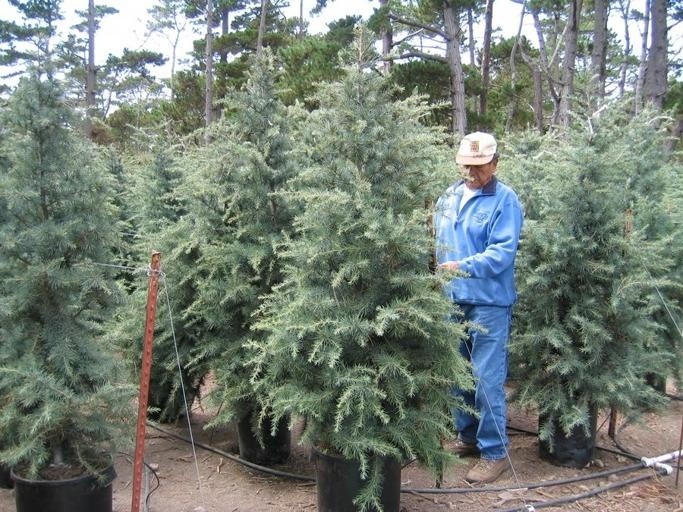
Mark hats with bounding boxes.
[454,131,497,166]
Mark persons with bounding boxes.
[427,131,522,486]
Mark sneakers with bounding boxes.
[443,441,480,457]
[466,457,514,485]
